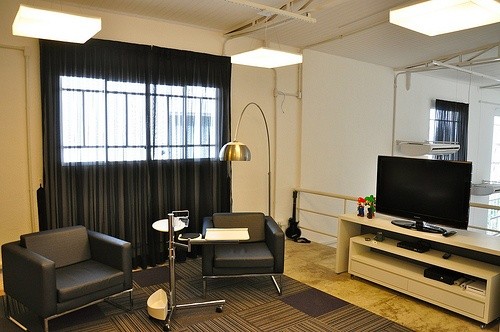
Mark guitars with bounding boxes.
[285,190,302,240]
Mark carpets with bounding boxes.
[0,258,423,332]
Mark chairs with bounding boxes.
[197,212,285,293]
[1,226,134,332]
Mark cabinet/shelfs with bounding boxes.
[347,234,500,328]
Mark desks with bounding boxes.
[334,211,500,321]
[159,228,251,332]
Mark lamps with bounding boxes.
[231,17,303,67]
[217,102,273,217]
[12,0,102,45]
[387,0,500,40]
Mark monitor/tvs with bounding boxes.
[375,155,471,236]
